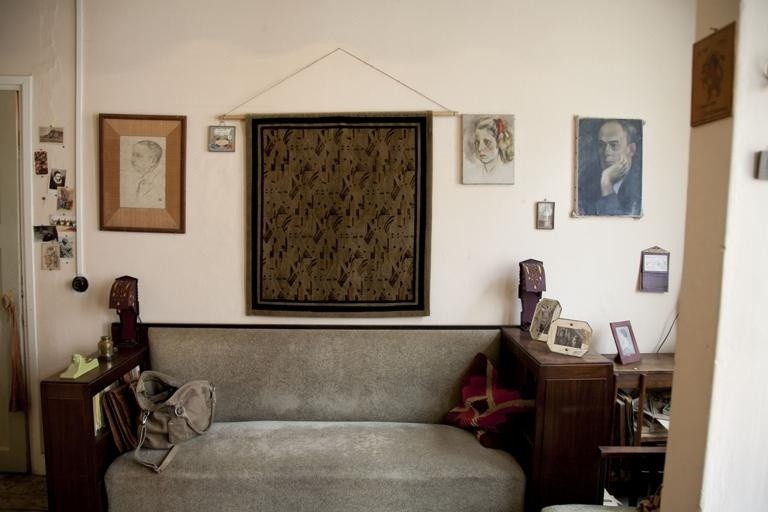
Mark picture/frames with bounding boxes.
[96,113,187,235]
[529,298,562,342]
[572,114,645,220]
[688,20,737,129]
[535,201,556,230]
[611,317,642,365]
[545,317,593,358]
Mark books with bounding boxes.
[616,388,670,446]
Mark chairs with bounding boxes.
[540,445,666,512]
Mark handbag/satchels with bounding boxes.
[134,369,216,470]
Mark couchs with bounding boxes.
[103,324,527,511]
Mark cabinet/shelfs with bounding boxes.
[38,341,152,512]
[601,353,675,496]
[500,328,614,511]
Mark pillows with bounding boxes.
[438,351,535,449]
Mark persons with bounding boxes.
[463,115,514,183]
[576,119,641,215]
[126,139,164,208]
[49,171,65,190]
[570,336,580,347]
[555,329,568,344]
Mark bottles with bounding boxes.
[98,335,113,359]
